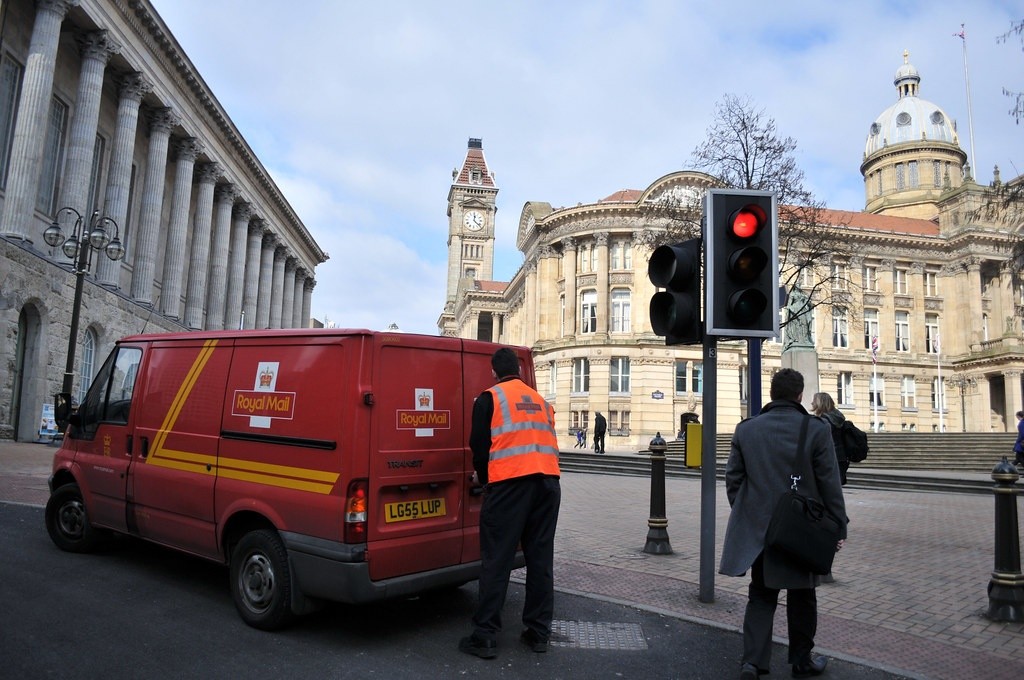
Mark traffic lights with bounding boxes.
[705,189,778,335]
[682,422,703,469]
[647,240,702,346]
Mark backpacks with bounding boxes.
[823,415,868,462]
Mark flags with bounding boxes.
[871,335,879,365]
[934,334,942,352]
[950,31,965,41]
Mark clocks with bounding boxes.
[462,209,487,231]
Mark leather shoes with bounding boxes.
[460,636,496,658]
[523,626,548,652]
[792,656,827,678]
[741,662,758,680]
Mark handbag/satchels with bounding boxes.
[767,493,842,574]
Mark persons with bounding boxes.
[809,392,850,528]
[1011,411,1024,469]
[593,409,606,454]
[459,348,561,658]
[718,370,850,680]
[574,426,587,450]
[782,282,816,355]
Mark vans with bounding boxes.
[43,327,527,633]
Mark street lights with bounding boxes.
[44,205,125,444]
